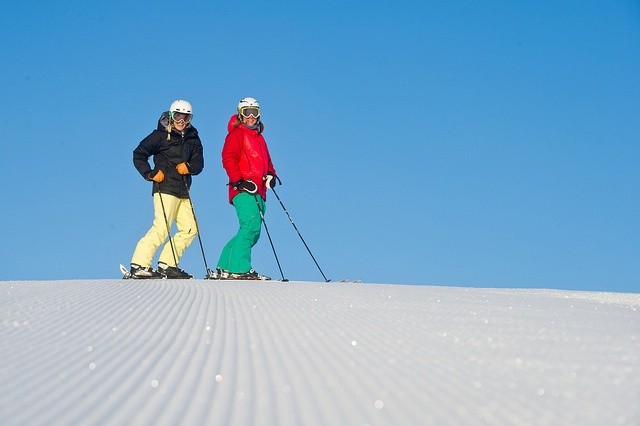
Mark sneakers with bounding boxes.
[216,267,229,277]
[157,262,192,277]
[231,268,270,280]
[130,263,159,277]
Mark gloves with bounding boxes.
[148,168,165,183]
[226,177,258,194]
[175,161,191,175]
[262,171,282,190]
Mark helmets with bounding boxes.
[170,99,192,114]
[237,97,259,111]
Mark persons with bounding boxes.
[216,97,276,278]
[130,100,203,279]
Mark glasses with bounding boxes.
[240,106,260,119]
[172,112,193,123]
